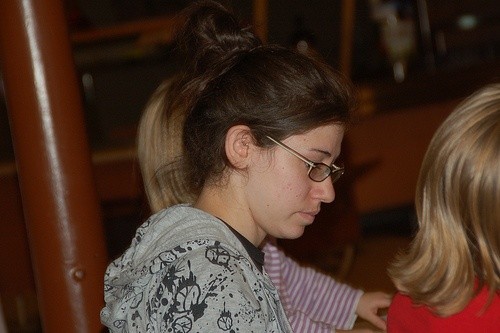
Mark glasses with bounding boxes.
[265,135,345,185]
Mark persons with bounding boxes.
[260,240,392,332]
[99,0,350,333]
[386,85,500,333]
